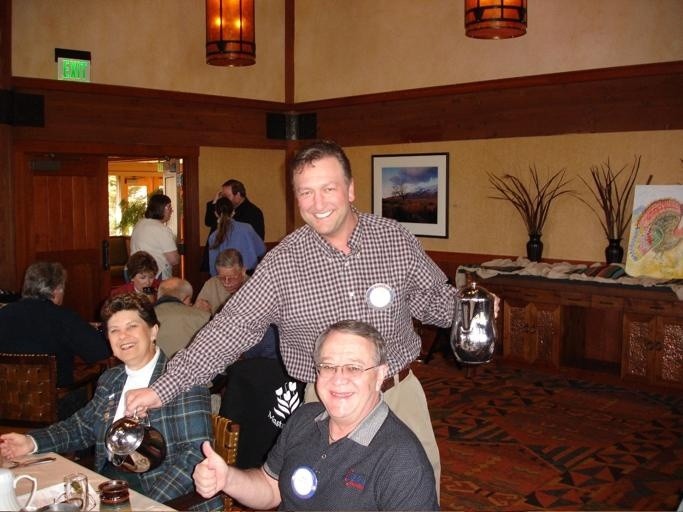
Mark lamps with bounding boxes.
[206,0,256,69]
[465,0,528,43]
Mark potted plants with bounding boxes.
[571,155,654,266]
[485,163,577,263]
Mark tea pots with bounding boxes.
[0,468,38,512]
[450,280,498,366]
[104,407,166,475]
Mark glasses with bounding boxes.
[312,362,379,375]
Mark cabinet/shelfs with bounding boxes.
[622,284,681,400]
[502,276,561,384]
[561,281,622,389]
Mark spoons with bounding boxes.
[3,455,56,470]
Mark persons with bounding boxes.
[130,195,181,280]
[195,249,276,360]
[213,323,307,470]
[101,252,159,319]
[124,138,500,511]
[149,278,214,358]
[192,319,440,511]
[0,292,226,511]
[208,198,268,276]
[204,178,265,249]
[0,262,113,387]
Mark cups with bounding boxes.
[64,473,96,512]
[177,241,186,255]
[98,480,132,512]
[142,286,158,295]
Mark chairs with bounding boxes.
[1,351,59,425]
[165,414,240,512]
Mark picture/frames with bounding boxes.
[370,152,450,239]
